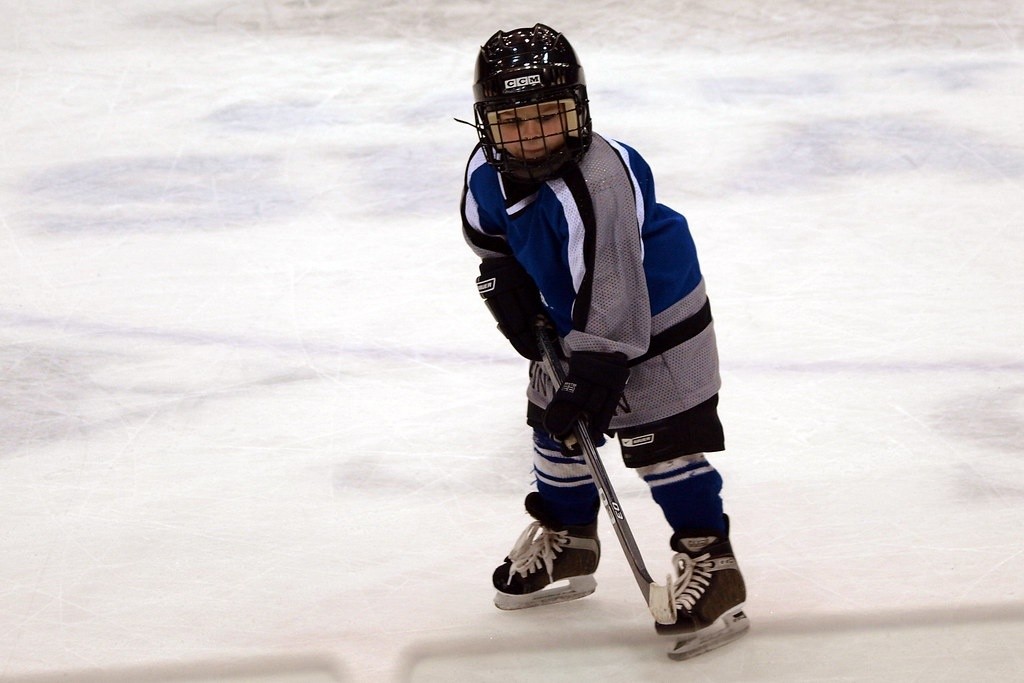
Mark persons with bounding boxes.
[453,22,749,661]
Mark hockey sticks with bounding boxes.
[535,326,679,627]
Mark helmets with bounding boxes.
[454,23,592,185]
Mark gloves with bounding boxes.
[476,255,558,361]
[542,351,631,457]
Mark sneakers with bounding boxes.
[655,513,750,660]
[491,492,601,610]
